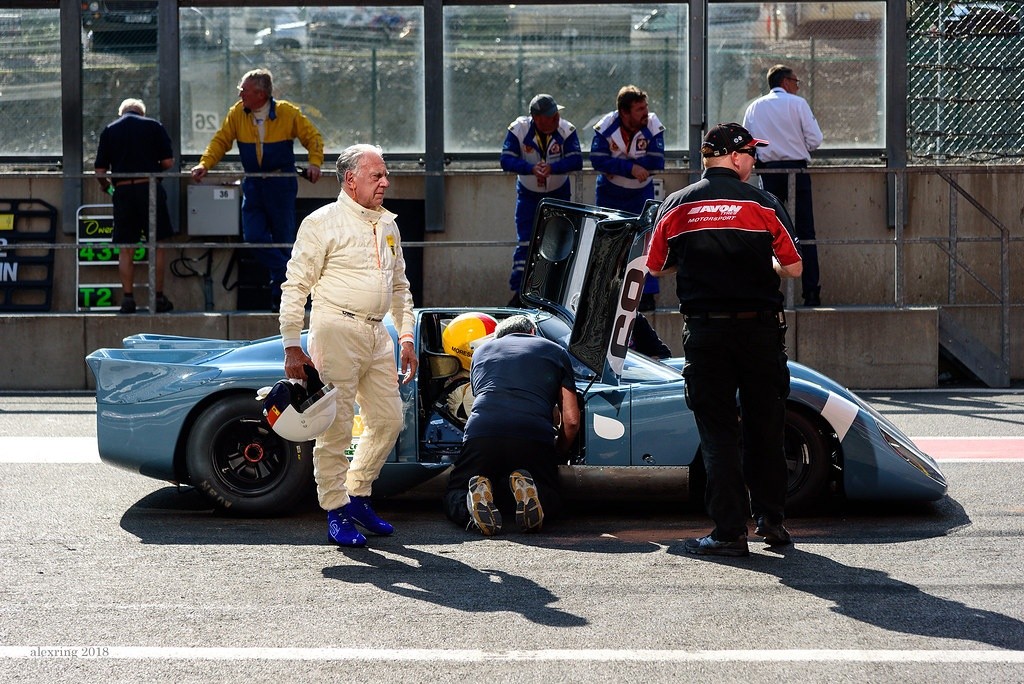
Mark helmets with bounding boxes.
[442,310,499,371]
[257,380,337,442]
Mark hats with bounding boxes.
[528,94,565,116]
[701,122,770,157]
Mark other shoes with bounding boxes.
[509,469,544,531]
[157,297,174,313]
[507,293,527,308]
[465,475,502,535]
[639,302,656,312]
[803,292,821,307]
[119,300,138,313]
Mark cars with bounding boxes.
[79,3,519,52]
[632,4,792,45]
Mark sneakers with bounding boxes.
[754,524,795,546]
[328,505,366,548]
[685,528,751,555]
[346,498,395,535]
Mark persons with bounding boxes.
[95,98,174,312]
[191,70,325,311]
[279,144,419,548]
[444,315,581,534]
[442,311,498,422]
[591,86,665,311]
[646,122,802,553]
[743,66,822,307]
[501,94,584,307]
[629,312,671,360]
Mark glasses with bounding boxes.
[786,77,801,85]
[737,148,758,160]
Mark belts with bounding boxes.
[342,311,382,322]
[687,311,759,320]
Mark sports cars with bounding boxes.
[86,199,949,519]
